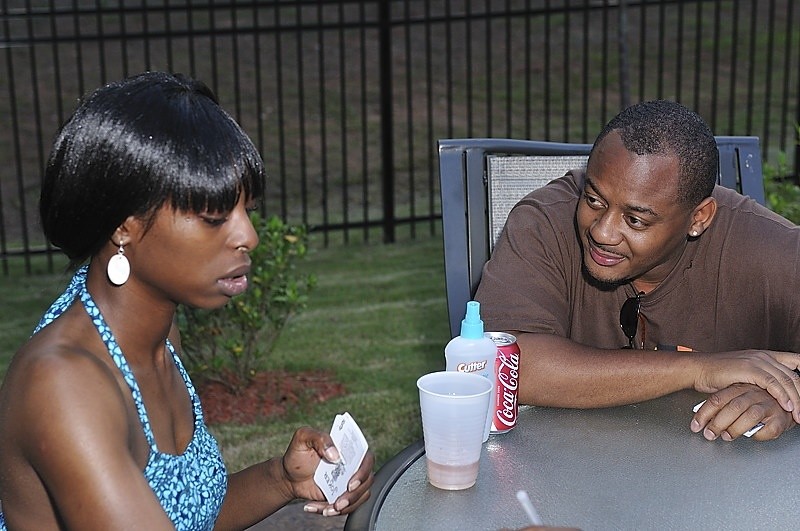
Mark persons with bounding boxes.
[0,73,374,531]
[471,100,800,441]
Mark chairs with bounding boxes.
[438,136,765,339]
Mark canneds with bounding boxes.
[482,332,520,435]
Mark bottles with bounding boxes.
[444,301,497,444]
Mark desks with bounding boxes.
[343,406,800,531]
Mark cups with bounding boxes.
[416,371,494,490]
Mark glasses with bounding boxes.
[619,291,644,350]
